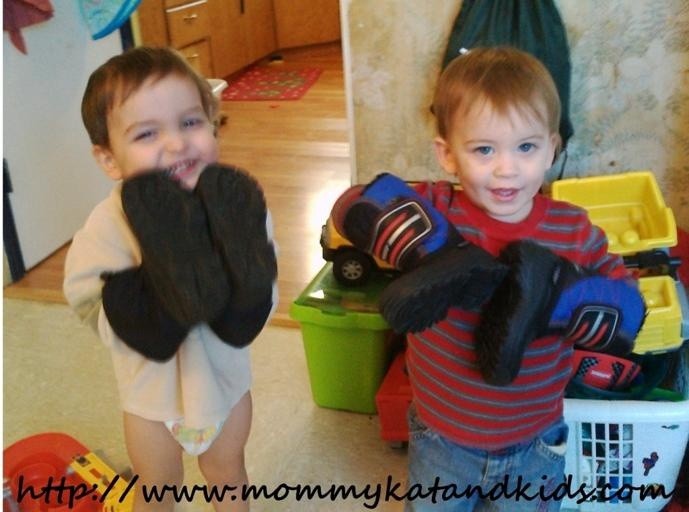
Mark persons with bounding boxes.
[63,43,278,512]
[332,44,646,511]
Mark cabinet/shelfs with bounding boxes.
[273,1,342,50]
[129,1,215,82]
[208,0,274,82]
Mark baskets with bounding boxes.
[556,246,689,512]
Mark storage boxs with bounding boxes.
[287,261,391,413]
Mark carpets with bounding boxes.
[221,64,325,100]
[4,296,410,512]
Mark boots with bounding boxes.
[99,167,232,364]
[342,172,510,336]
[472,238,647,387]
[193,160,278,349]
